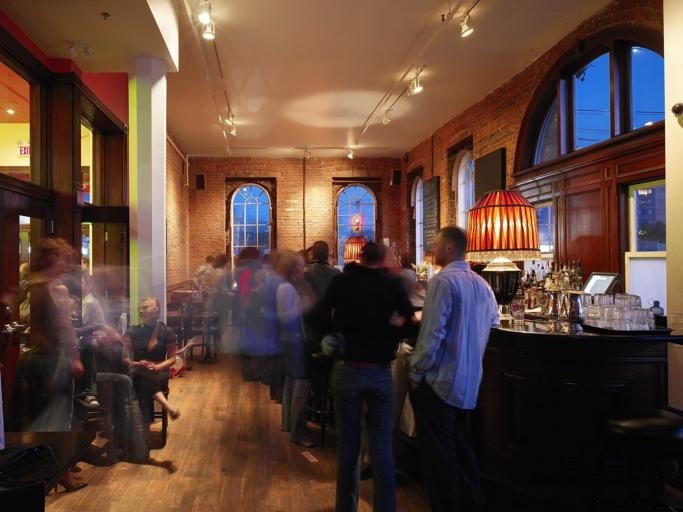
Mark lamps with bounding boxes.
[225,112,237,137]
[199,3,215,39]
[463,190,542,326]
[460,21,473,39]
[343,235,369,264]
[380,77,423,126]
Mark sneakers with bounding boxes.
[294,435,315,448]
[171,409,181,420]
[361,460,372,481]
[199,354,213,364]
[77,395,99,409]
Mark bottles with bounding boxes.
[650,300,664,315]
[522,257,583,292]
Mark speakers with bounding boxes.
[391,169,401,186]
[195,173,206,192]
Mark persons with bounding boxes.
[193,241,343,449]
[305,241,423,510]
[404,227,500,510]
[22,239,179,495]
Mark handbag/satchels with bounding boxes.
[0,442,57,487]
[320,332,344,358]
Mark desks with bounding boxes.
[171,290,235,339]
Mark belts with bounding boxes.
[350,361,383,368]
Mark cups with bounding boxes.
[511,304,524,327]
[579,293,655,331]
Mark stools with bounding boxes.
[305,352,346,450]
[606,406,682,512]
[151,386,171,447]
[167,301,220,362]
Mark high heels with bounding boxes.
[54,476,88,493]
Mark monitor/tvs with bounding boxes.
[580,271,624,299]
